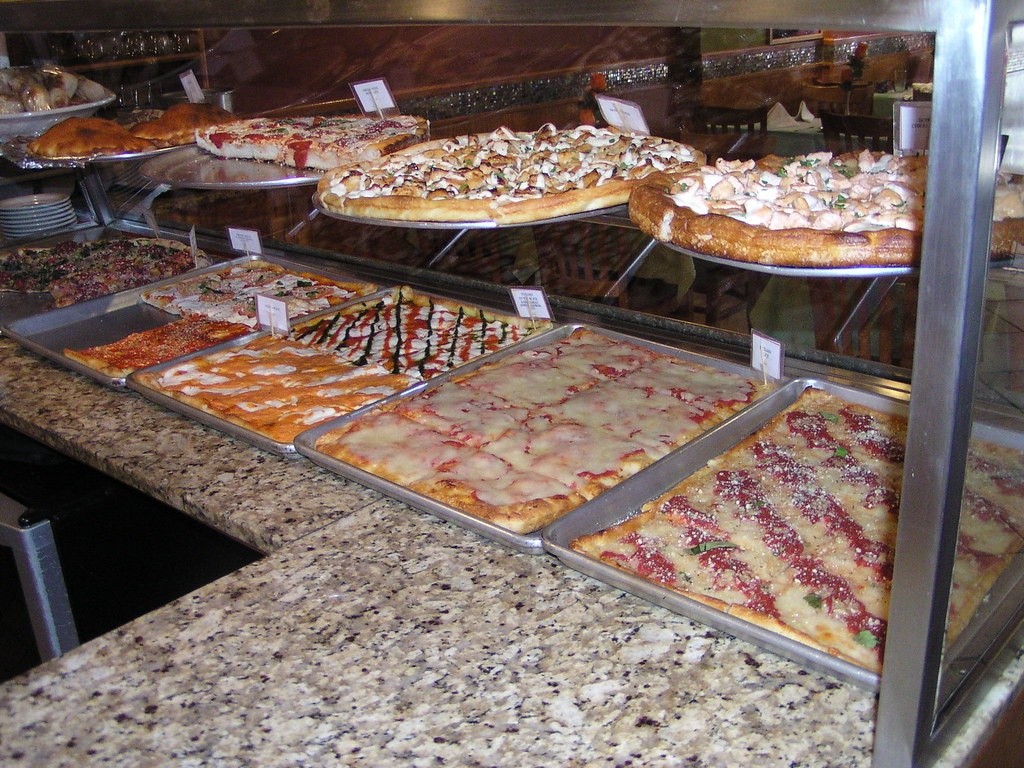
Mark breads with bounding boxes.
[0,65,106,115]
[29,102,240,157]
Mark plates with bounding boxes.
[1,194,77,242]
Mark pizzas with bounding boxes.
[59,259,555,443]
[310,326,768,537]
[629,150,1024,267]
[570,385,1024,680]
[193,114,431,171]
[0,236,211,307]
[314,124,706,225]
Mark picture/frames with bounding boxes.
[767,29,824,46]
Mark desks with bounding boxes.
[653,129,919,168]
[872,85,915,117]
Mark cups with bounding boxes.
[894,68,908,94]
[52,24,197,64]
[122,81,163,112]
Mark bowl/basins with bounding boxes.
[163,88,235,121]
[1,66,116,138]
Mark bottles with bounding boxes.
[822,38,835,62]
[822,65,831,83]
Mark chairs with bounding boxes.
[528,78,1008,371]
